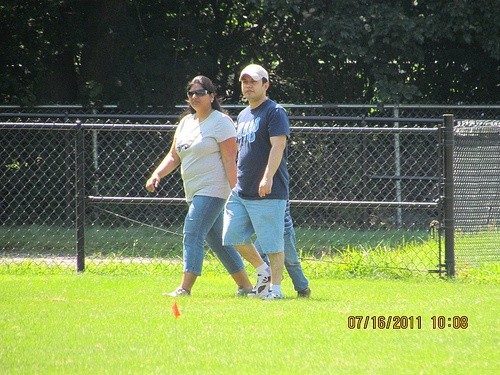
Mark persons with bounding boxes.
[145,76,255,300]
[220,64,290,301]
[254,198,312,298]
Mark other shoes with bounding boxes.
[297,286,311,297]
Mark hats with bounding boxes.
[238,63,269,81]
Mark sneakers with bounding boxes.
[235,287,254,297]
[247,273,285,301]
[163,287,190,298]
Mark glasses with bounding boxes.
[187,89,211,96]
